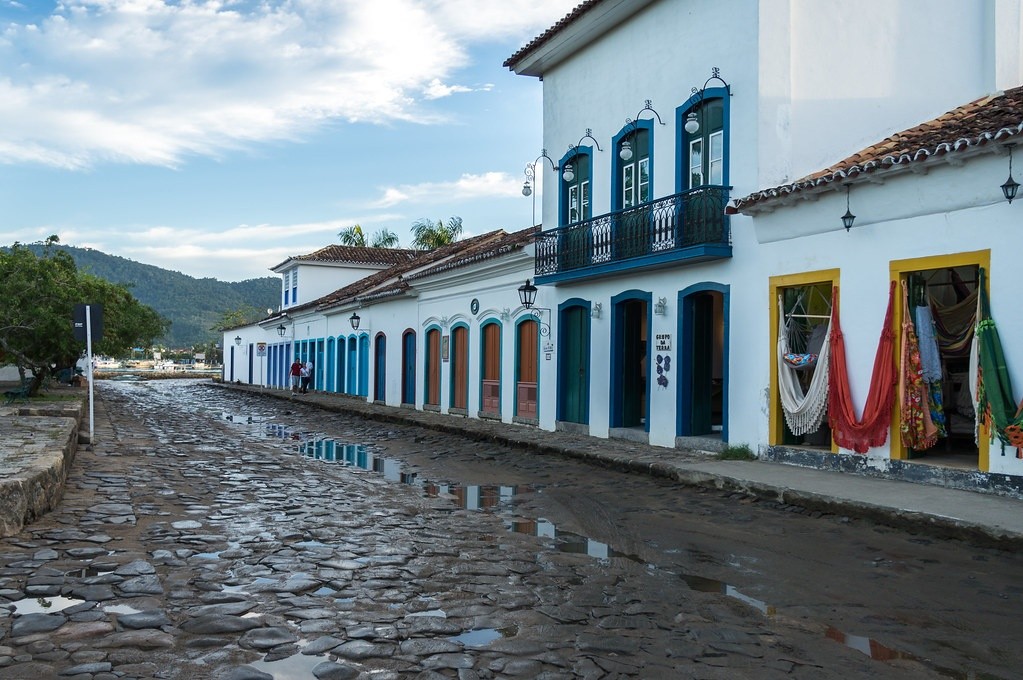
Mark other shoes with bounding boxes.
[303,393,307,396]
[292,392,297,396]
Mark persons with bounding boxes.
[288,358,312,396]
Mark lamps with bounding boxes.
[590,301,603,320]
[620,100,666,160]
[840,183,856,232]
[349,312,371,346]
[521,148,560,196]
[1000,142,1021,204]
[517,279,551,340]
[499,307,510,322]
[234,336,248,355]
[654,297,668,317]
[684,66,733,134]
[276,324,295,340]
[563,128,603,182]
[438,315,448,328]
